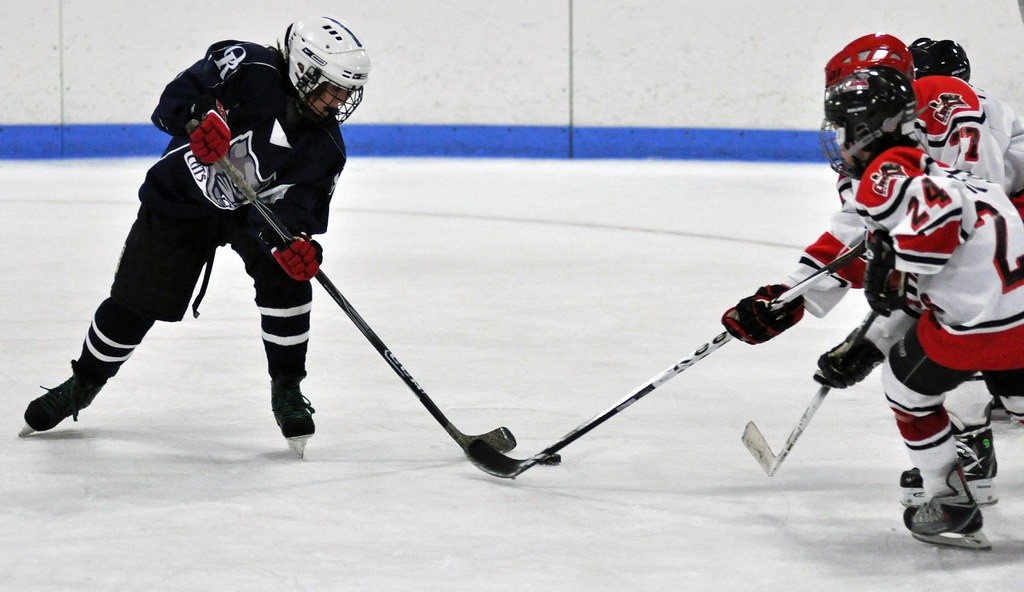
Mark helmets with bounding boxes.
[821,65,918,181]
[284,16,370,128]
[907,37,971,82]
[824,33,914,90]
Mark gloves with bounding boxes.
[813,327,886,389]
[257,229,323,282]
[863,229,896,318]
[185,92,232,166]
[721,284,806,345]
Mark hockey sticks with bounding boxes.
[465,233,883,481]
[739,269,902,478]
[183,118,518,458]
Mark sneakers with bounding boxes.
[18,377,91,438]
[903,457,992,551]
[980,394,1024,431]
[900,417,998,509]
[271,379,315,459]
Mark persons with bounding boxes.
[814,62,1024,549]
[722,34,1023,508]
[20,16,370,458]
